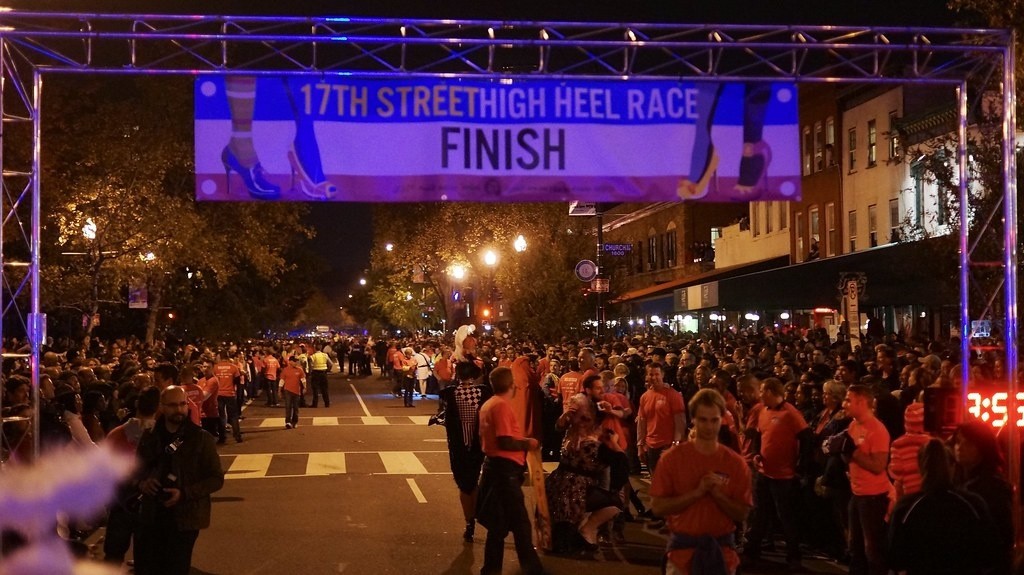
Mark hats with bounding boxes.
[648,347,665,356]
[148,363,178,378]
[917,355,942,369]
[290,356,299,362]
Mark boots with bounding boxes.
[629,492,645,513]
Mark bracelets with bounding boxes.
[672,440,681,445]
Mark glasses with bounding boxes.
[162,402,189,407]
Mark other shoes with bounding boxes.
[578,532,598,551]
[789,559,800,570]
[624,510,633,522]
[285,422,293,428]
[309,405,317,408]
[216,440,225,444]
[659,525,670,534]
[325,401,330,407]
[463,523,475,542]
[742,556,759,566]
[644,521,662,530]
[234,436,242,442]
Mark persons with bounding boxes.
[0,311,1024,575]
